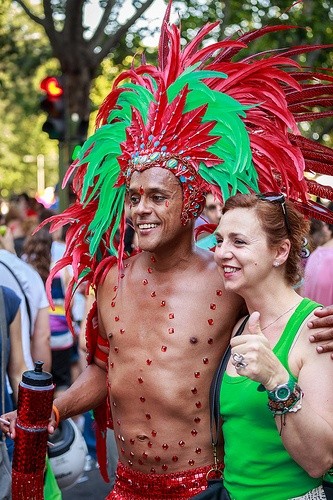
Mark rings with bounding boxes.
[233,353,246,369]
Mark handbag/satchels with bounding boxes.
[0,442,13,500]
[190,479,231,500]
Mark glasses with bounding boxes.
[255,192,291,239]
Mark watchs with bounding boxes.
[266,376,295,401]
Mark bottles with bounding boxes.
[10,361,55,499]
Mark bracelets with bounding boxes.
[53,406,59,428]
[268,384,304,436]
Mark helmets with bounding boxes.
[47,417,88,489]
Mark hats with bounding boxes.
[30,0,333,335]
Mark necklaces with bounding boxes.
[261,303,300,331]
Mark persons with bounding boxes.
[212,194,333,500]
[301,237,333,307]
[0,154,333,500]
[1,183,99,500]
[195,194,223,248]
